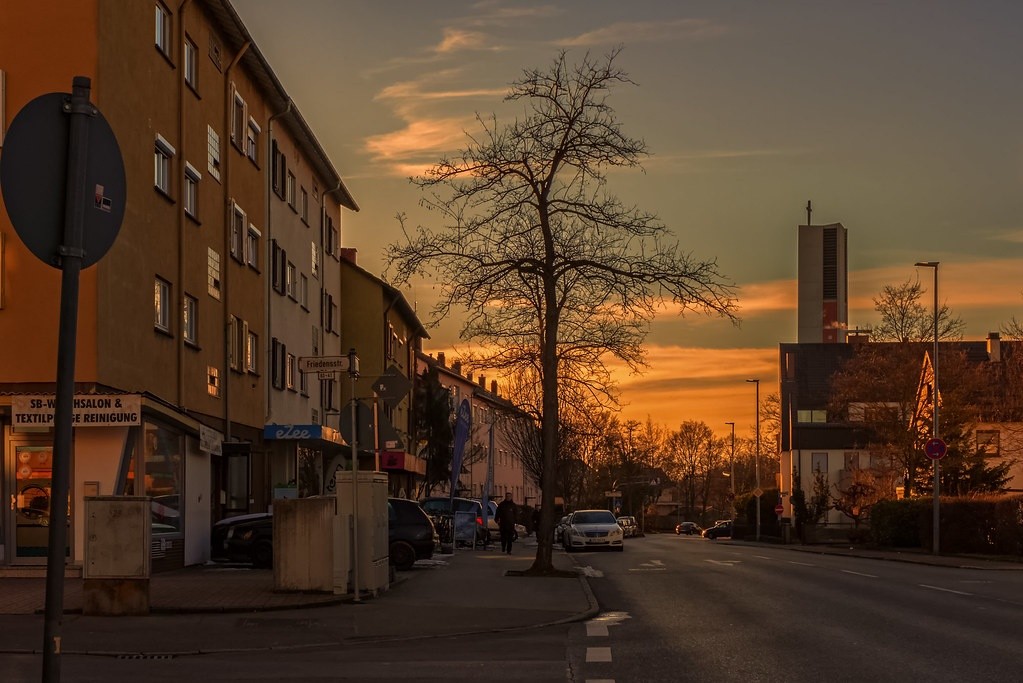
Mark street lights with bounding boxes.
[611,477,660,518]
[745,378,764,542]
[724,421,735,526]
[914,260,942,556]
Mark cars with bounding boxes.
[557,516,567,544]
[561,513,573,547]
[417,495,488,548]
[486,500,528,544]
[211,493,441,573]
[564,509,625,551]
[702,520,731,540]
[676,522,702,536]
[616,516,639,538]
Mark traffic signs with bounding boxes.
[354,356,360,382]
[297,355,351,374]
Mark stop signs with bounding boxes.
[775,504,784,515]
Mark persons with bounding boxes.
[530,509,540,543]
[493,492,520,556]
[792,506,803,542]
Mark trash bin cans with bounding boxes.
[801,518,816,545]
